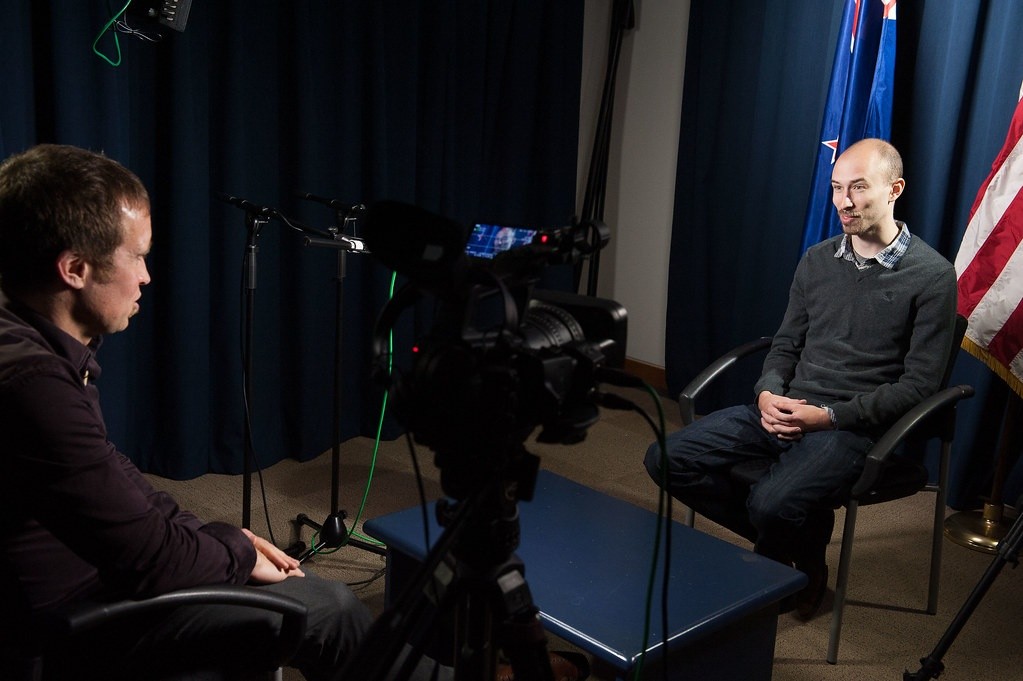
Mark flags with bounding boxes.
[953,80,1023,398]
[802,1,897,254]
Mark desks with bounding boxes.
[363,466,808,681]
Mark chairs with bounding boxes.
[0,585,311,681]
[679,313,974,665]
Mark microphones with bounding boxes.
[298,235,370,256]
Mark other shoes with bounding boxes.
[753,545,827,622]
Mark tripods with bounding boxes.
[308,495,557,681]
[295,247,393,570]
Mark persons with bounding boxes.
[0,144,368,681]
[643,137,958,625]
[478,227,516,259]
